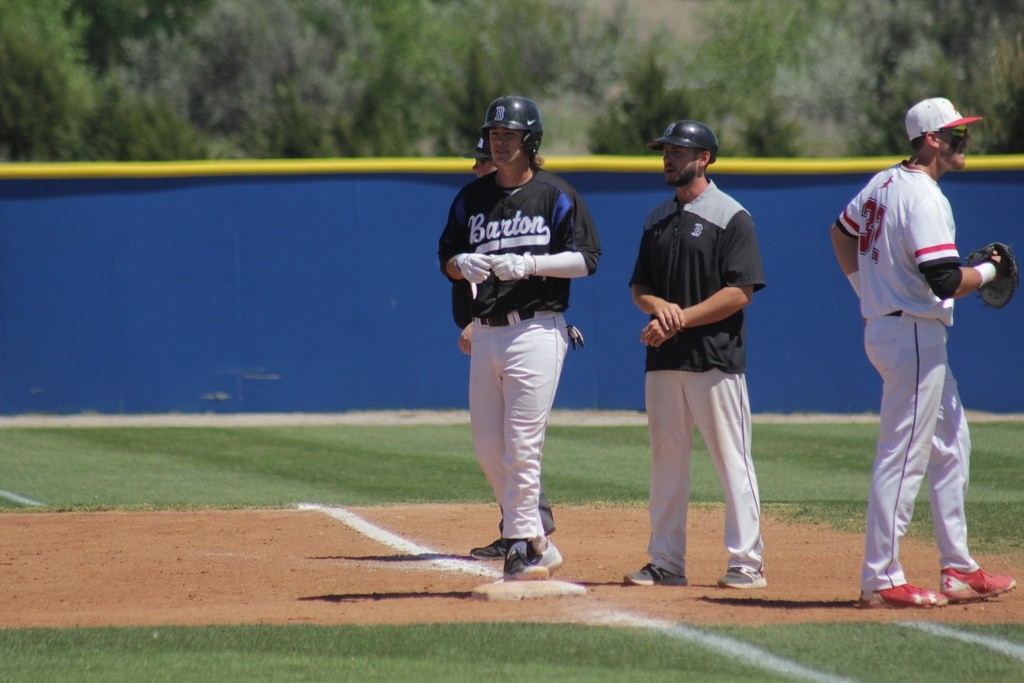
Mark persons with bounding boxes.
[830,96,1018,611]
[437,95,603,583]
[623,120,768,591]
[450,128,555,539]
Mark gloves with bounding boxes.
[566,324,585,349]
[487,253,536,280]
[455,253,495,284]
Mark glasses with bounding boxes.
[923,125,968,139]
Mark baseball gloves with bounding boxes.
[966,242,1021,310]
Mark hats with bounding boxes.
[462,136,492,159]
[905,98,984,142]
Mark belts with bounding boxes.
[888,310,902,317]
[472,311,534,328]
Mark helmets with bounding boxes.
[479,96,542,154]
[647,121,718,163]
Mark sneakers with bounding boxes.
[623,564,687,587]
[496,539,562,585]
[938,564,1017,605]
[859,577,947,608]
[718,563,768,589]
[469,538,506,560]
